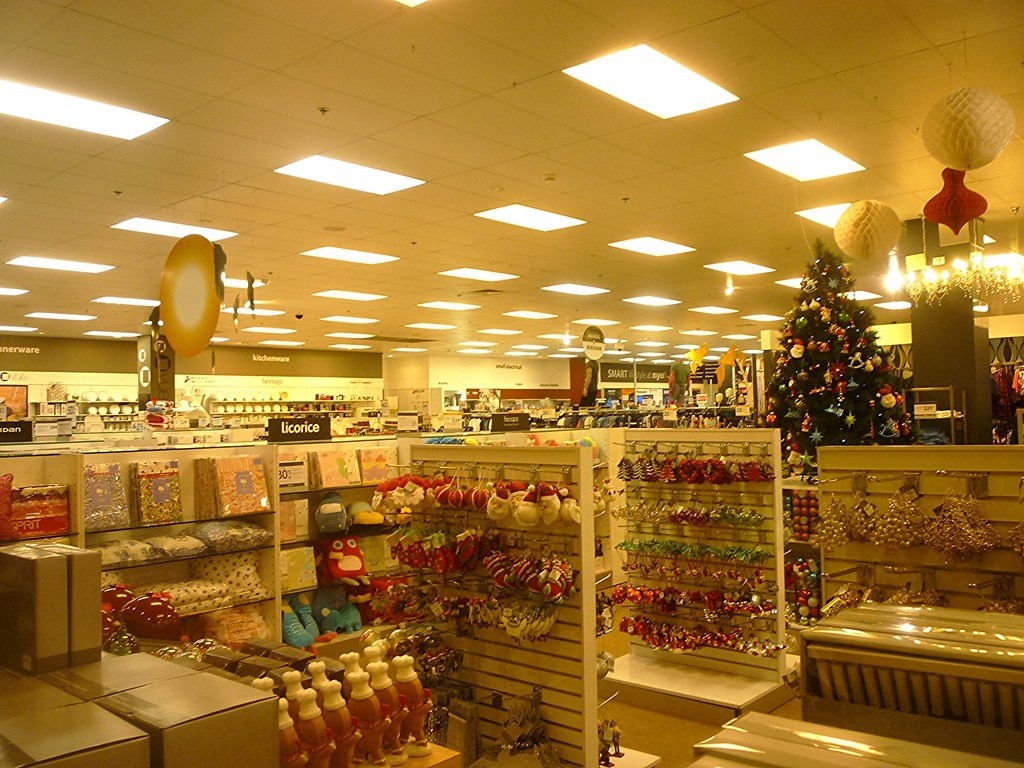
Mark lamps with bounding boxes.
[906,236,1023,313]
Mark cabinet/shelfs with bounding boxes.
[0,451,82,549]
[782,476,822,655]
[277,438,411,666]
[897,378,974,447]
[80,445,279,658]
[31,394,537,441]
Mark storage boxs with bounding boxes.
[685,706,1024,768]
[0,539,280,768]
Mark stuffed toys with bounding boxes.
[281,473,616,659]
